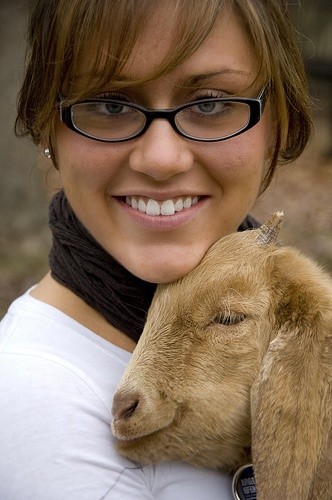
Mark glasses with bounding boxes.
[56,80,272,143]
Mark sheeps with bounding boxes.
[109,209,332,500]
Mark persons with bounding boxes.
[0,0,315,500]
